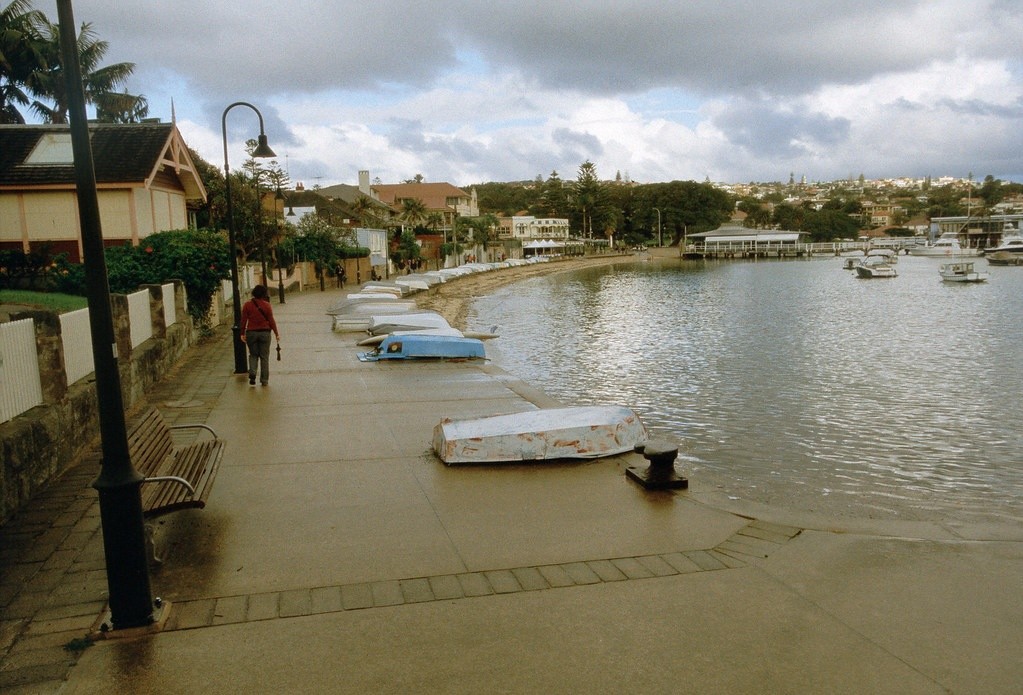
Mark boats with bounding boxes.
[854,255,899,278]
[321,257,549,363]
[866,249,899,264]
[982,237,1023,256]
[430,404,652,463]
[907,237,984,257]
[938,260,989,283]
[843,252,867,270]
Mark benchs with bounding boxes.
[128,405,227,568]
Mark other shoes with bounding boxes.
[262,382,268,386]
[248,372,256,385]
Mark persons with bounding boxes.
[398,258,416,275]
[498,251,507,261]
[241,285,280,385]
[335,264,344,289]
[371,265,377,281]
[464,253,476,264]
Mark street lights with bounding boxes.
[222,101,273,378]
[274,194,297,303]
[315,208,334,290]
[256,168,288,303]
[652,207,662,247]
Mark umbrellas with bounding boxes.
[523,239,561,256]
